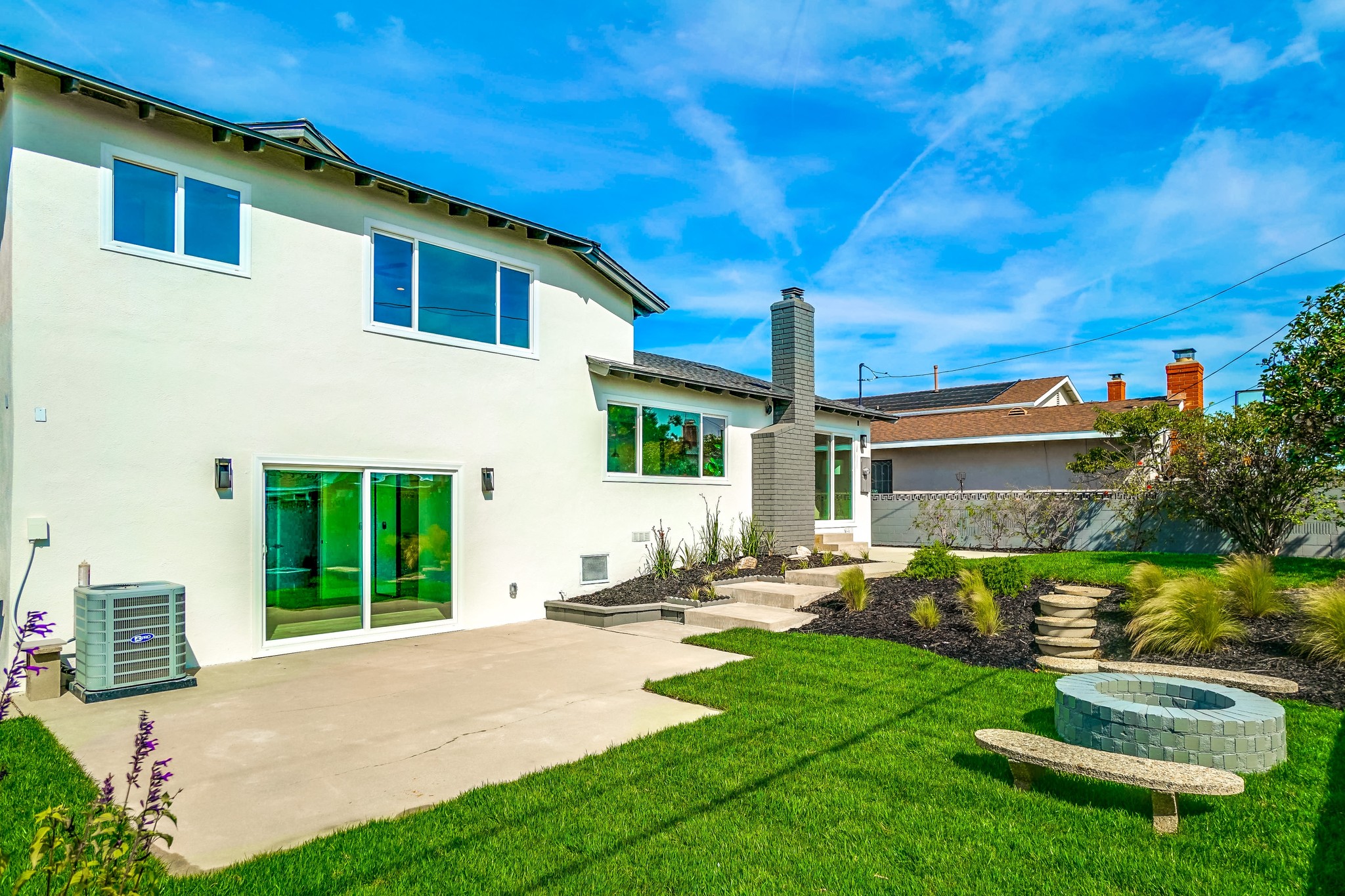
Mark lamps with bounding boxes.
[510,582,518,595]
[860,434,867,449]
[214,457,233,489]
[481,467,494,491]
[766,405,773,414]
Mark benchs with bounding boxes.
[975,729,1246,834]
[1097,661,1299,693]
